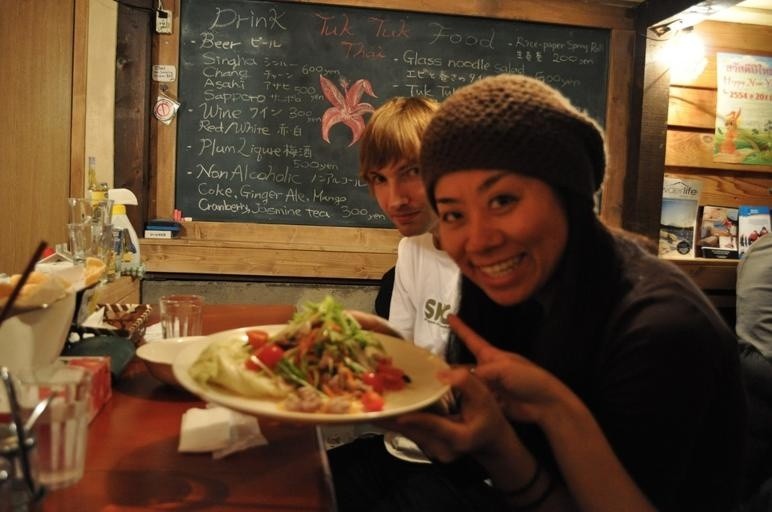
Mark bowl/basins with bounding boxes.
[135,335,205,386]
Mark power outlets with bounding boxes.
[154,8,175,34]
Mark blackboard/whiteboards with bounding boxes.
[157,0,635,255]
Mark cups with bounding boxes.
[68,183,125,285]
[16,365,92,485]
[0,423,41,512]
[159,292,206,339]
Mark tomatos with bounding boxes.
[362,355,410,412]
[244,329,285,371]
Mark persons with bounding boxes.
[734,227,772,360]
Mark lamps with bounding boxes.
[665,24,711,87]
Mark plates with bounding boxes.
[173,323,452,426]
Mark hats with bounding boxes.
[420,74,606,216]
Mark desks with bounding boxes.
[28,300,338,511]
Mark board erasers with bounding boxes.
[147,219,182,232]
